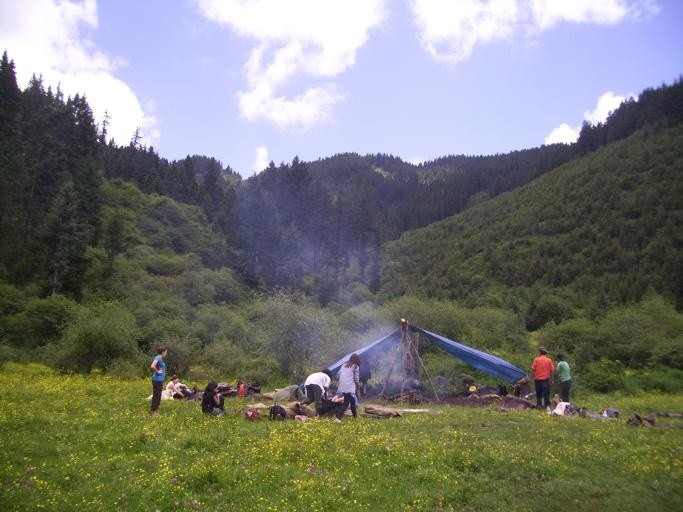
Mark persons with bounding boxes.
[529,347,554,408]
[555,353,570,403]
[300,369,332,420]
[200,381,225,415]
[333,393,358,409]
[150,346,167,417]
[335,353,360,422]
[167,375,192,399]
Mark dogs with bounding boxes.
[268,401,287,421]
